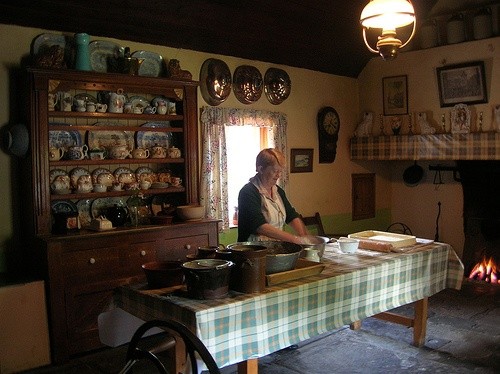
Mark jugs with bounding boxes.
[108,93,125,113]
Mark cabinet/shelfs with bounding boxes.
[8,65,224,365]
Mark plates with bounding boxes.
[73,91,171,109]
[89,41,124,73]
[33,33,75,70]
[50,193,177,231]
[51,168,155,186]
[49,122,174,147]
[131,50,163,77]
[152,183,168,189]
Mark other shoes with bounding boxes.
[284,345,298,350]
[276,349,283,355]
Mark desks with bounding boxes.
[112,231,465,374]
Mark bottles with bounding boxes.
[74,33,92,71]
[197,246,220,259]
[157,102,167,114]
[304,248,321,262]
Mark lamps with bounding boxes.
[360,0,417,62]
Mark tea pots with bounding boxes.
[100,205,129,227]
[124,103,143,114]
[144,105,156,114]
[49,146,181,161]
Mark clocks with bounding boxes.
[317,106,341,164]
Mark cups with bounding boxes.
[76,101,107,112]
[170,177,182,186]
[125,181,152,190]
[49,94,73,111]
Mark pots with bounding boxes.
[404,161,424,183]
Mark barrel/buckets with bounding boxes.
[184,245,267,300]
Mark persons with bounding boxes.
[237,148,318,351]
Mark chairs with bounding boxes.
[299,211,347,239]
[128,320,220,374]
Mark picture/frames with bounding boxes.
[290,148,314,173]
[382,74,409,116]
[436,61,488,108]
[352,173,375,221]
[450,103,470,134]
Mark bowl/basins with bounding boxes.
[176,205,206,222]
[142,254,197,290]
[227,237,360,275]
